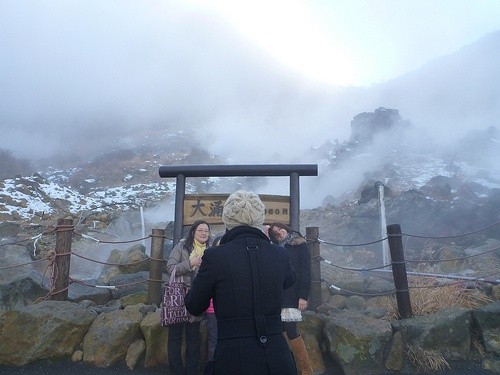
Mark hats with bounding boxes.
[222,190,265,231]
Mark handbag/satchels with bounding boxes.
[160,265,192,327]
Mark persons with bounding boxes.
[184,189,297,375]
[268,222,314,375]
[204,236,222,361]
[165,219,211,375]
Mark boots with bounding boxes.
[282,331,313,375]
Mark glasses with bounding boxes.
[196,230,209,233]
